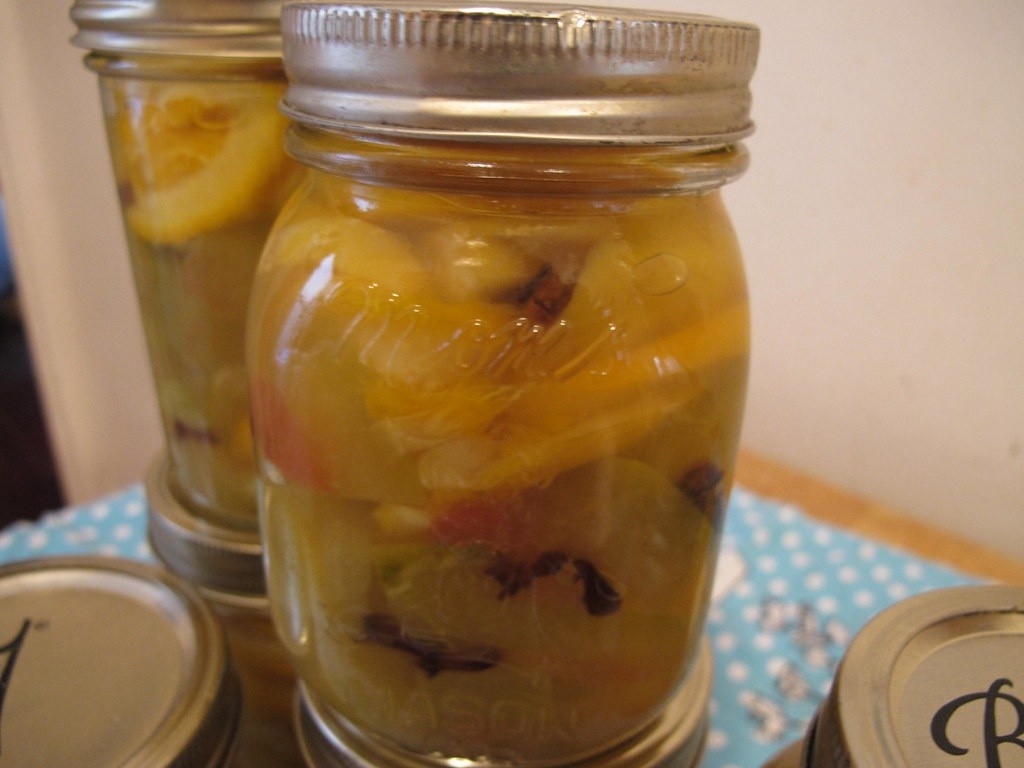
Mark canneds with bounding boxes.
[1,0,1024,768]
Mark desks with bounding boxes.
[0,449,1023,767]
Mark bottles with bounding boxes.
[241,1,762,768]
[66,0,310,542]
[139,445,302,768]
[0,554,247,768]
[760,582,1024,767]
